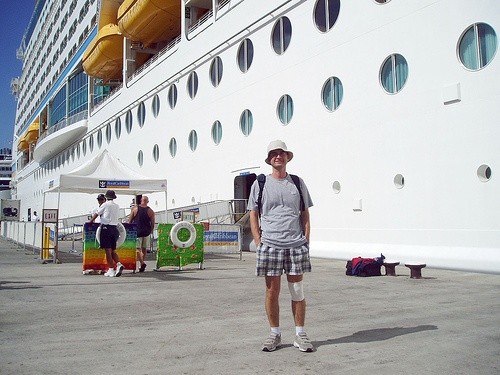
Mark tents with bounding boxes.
[41,149,168,263]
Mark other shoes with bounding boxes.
[139,264,147,273]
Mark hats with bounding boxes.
[96,194,105,199]
[265,140,293,163]
[104,190,117,199]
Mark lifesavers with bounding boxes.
[96,222,126,249]
[170,221,197,248]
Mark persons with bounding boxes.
[32,211,40,221]
[130,199,135,212]
[127,196,155,273]
[85,191,124,277]
[92,194,106,224]
[249,141,313,352]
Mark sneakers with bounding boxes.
[262,332,282,352]
[104,270,115,277]
[294,332,313,352]
[115,264,124,277]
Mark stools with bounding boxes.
[383,261,400,275]
[405,264,426,279]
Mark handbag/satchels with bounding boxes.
[353,257,377,268]
[346,260,352,275]
[353,256,386,276]
